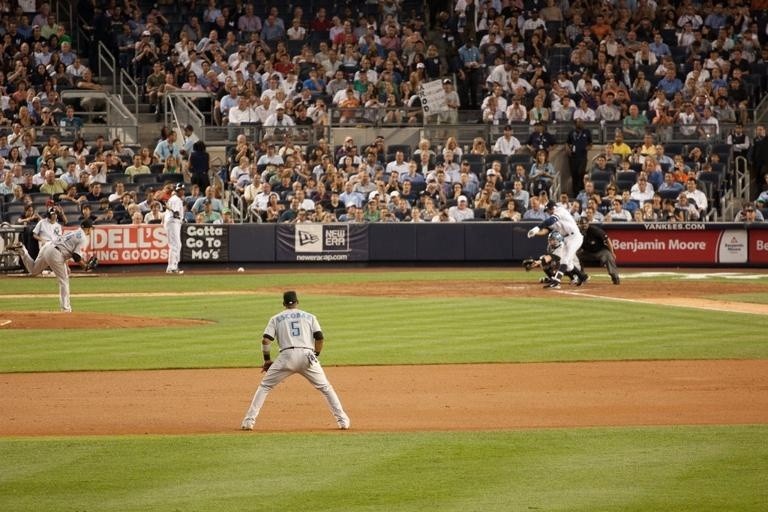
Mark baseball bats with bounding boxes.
[158,198,175,213]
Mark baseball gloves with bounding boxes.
[83,255,98,272]
[523,258,534,270]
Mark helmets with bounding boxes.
[174,182,186,192]
[548,231,563,242]
[576,216,590,235]
[48,207,56,215]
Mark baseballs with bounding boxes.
[238,267,244,273]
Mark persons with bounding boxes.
[2,1,767,222]
[7,208,95,312]
[240,290,351,430]
[160,183,187,275]
[525,201,619,289]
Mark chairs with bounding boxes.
[0,0,768,226]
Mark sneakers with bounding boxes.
[538,273,588,289]
[166,269,184,274]
[5,242,22,251]
[611,278,620,285]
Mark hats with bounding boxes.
[80,219,95,230]
[202,199,211,205]
[543,201,556,212]
[221,207,231,214]
[284,291,297,305]
[457,195,467,203]
[487,168,496,176]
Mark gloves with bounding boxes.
[173,210,179,219]
[528,226,540,239]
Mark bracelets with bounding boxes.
[315,351,320,357]
[261,344,272,352]
[264,354,271,361]
